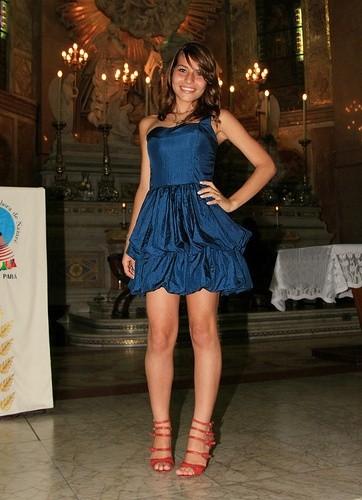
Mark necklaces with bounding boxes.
[169,107,195,124]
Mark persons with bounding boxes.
[122,42,277,480]
[120,104,136,140]
[254,91,267,138]
[107,23,127,67]
[61,74,78,135]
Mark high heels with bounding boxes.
[149,419,175,473]
[175,416,217,479]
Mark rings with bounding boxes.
[213,193,216,198]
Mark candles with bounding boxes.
[122,201,126,227]
[275,205,279,229]
[56,43,307,139]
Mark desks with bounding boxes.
[269,243,362,326]
